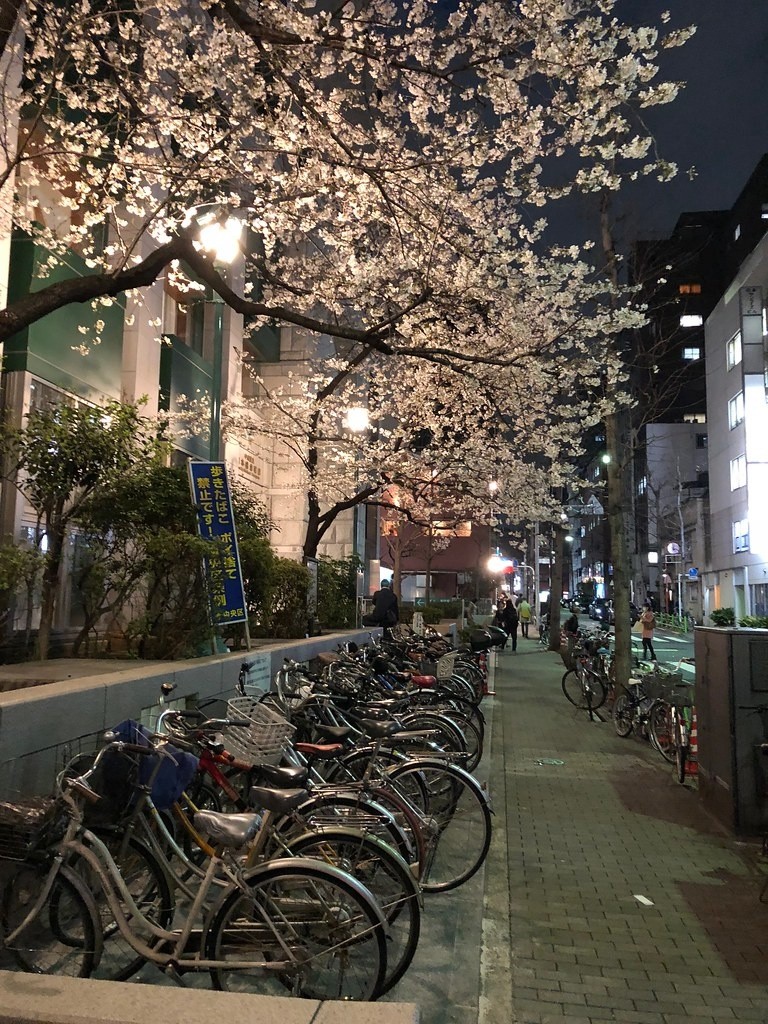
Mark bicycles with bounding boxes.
[0,620,510,1003]
[559,626,696,785]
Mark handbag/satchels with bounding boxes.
[644,614,657,630]
[105,719,192,807]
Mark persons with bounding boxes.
[371,579,400,624]
[490,590,612,652]
[639,603,658,661]
[644,596,685,616]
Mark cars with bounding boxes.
[607,600,640,627]
[588,598,612,622]
[570,593,596,614]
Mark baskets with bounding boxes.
[0,757,67,862]
[643,671,694,706]
[60,733,138,821]
[220,695,295,766]
[185,690,228,730]
[559,646,579,671]
